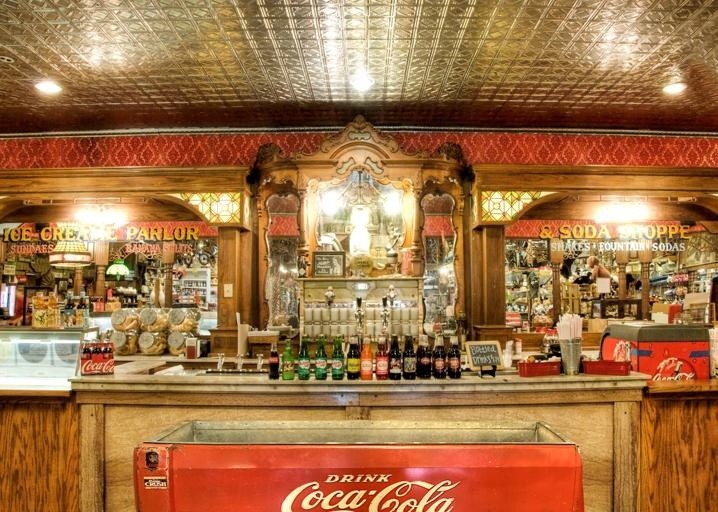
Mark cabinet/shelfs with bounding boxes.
[1,325,100,397]
[294,276,423,352]
[182,268,211,309]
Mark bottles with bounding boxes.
[331,334,344,380]
[184,281,206,287]
[552,330,560,354]
[282,339,295,380]
[360,337,373,380]
[138,332,167,356]
[167,304,200,332]
[376,338,388,380]
[298,335,310,380]
[416,334,431,378]
[110,333,137,355]
[268,341,279,380]
[314,334,328,380]
[76,292,86,327]
[432,335,446,379]
[140,307,168,332]
[389,334,402,380]
[47,292,60,327]
[346,338,361,380]
[34,292,46,327]
[166,333,194,355]
[515,338,522,355]
[91,339,103,375]
[81,339,91,376]
[403,334,416,380]
[447,335,461,379]
[109,309,139,331]
[63,291,76,328]
[102,339,114,374]
[543,331,552,353]
[91,295,104,312]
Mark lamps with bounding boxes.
[48,239,91,269]
[106,260,129,281]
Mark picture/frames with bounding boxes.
[311,251,346,277]
[464,340,505,372]
